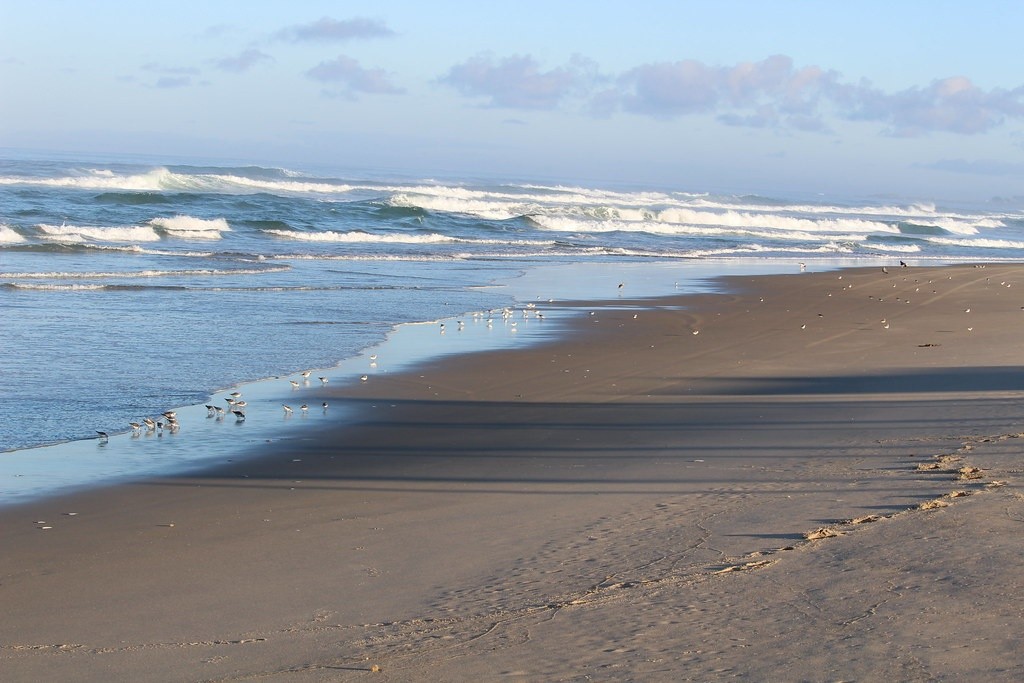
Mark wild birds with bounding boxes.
[759,260,1011,330]
[589,282,700,336]
[440,295,553,330]
[93,354,378,440]
